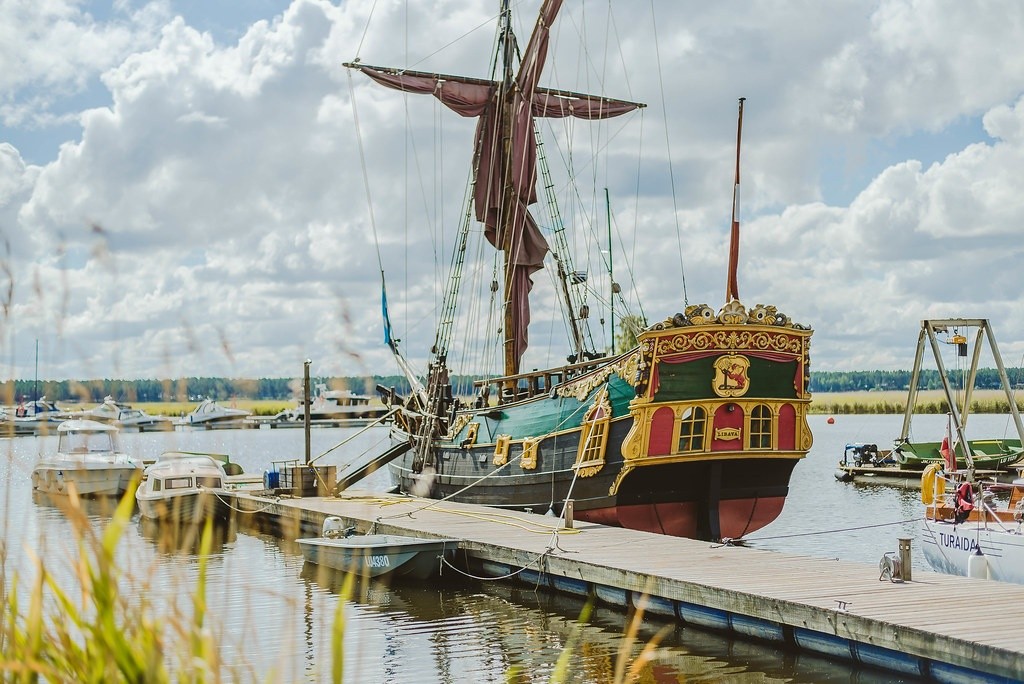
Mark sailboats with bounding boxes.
[341,0,817,546]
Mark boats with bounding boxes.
[921,463,1024,584]
[135,453,237,525]
[26,419,144,497]
[293,517,462,582]
[0,385,388,435]
[834,438,1024,492]
